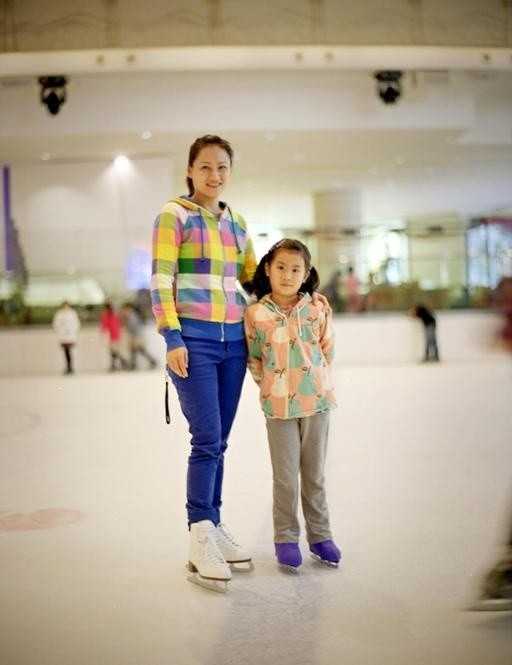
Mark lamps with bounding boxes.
[38,74,68,116]
[375,69,401,103]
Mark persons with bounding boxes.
[247,236,344,568]
[53,301,81,374]
[150,143,330,583]
[120,303,155,370]
[328,272,342,311]
[345,265,362,311]
[406,304,441,362]
[100,302,131,371]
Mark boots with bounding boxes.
[185,520,251,581]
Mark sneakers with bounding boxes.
[309,540,341,563]
[275,542,302,567]
